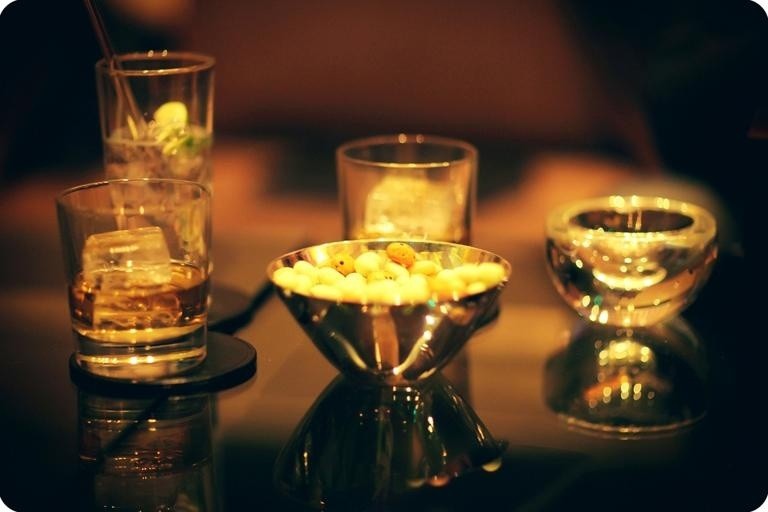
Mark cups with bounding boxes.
[335,135,477,239]
[546,199,721,329]
[67,395,211,512]
[542,327,716,441]
[96,51,216,176]
[53,177,211,380]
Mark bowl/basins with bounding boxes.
[278,376,507,507]
[264,242,511,377]
